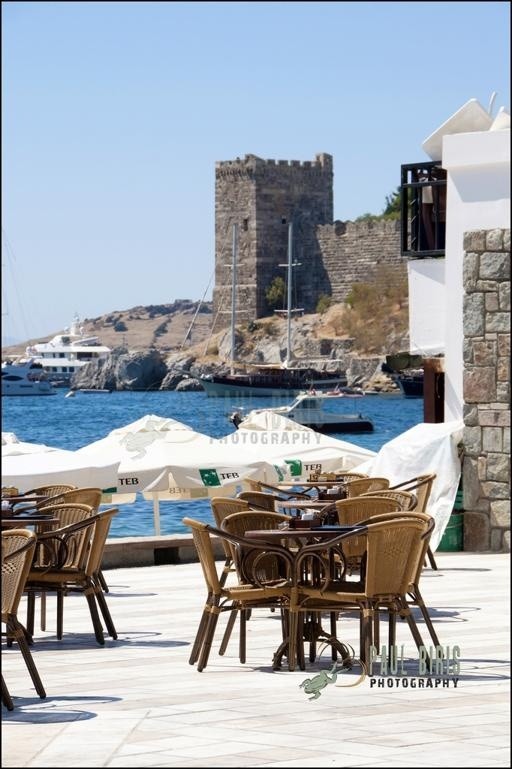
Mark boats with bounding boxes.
[228,389,375,434]
[2,316,113,400]
[388,371,423,398]
[165,221,347,398]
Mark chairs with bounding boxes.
[2,480,120,712]
[182,469,443,680]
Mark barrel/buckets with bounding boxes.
[435,513,463,552]
